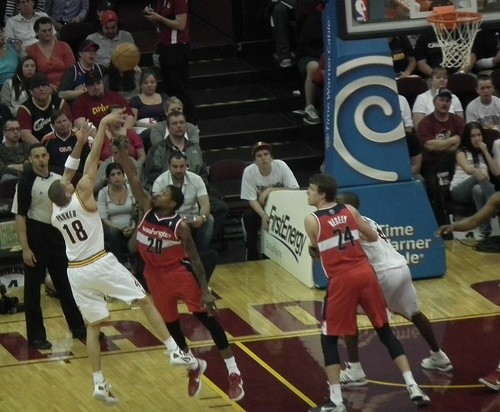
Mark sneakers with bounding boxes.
[169,347,196,367]
[188,359,206,398]
[228,372,244,401]
[420,357,452,371]
[479,375,500,391]
[406,385,431,405]
[93,380,118,407]
[327,370,368,387]
[303,105,320,124]
[308,400,346,412]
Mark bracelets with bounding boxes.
[202,215,206,222]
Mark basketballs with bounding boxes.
[112,42,139,70]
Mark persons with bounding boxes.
[435,190,500,390]
[240,141,299,260]
[265,0,323,124]
[381,0,500,238]
[110,133,244,400]
[336,192,451,386]
[11,143,104,349]
[303,174,430,412]
[49,109,199,407]
[0,0,229,263]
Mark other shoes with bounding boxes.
[27,339,52,349]
[73,327,104,338]
[476,230,491,242]
[279,59,293,67]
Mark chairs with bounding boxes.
[433,160,476,239]
[396,74,426,101]
[204,159,254,248]
[445,72,476,105]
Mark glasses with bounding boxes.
[4,127,20,132]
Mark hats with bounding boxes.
[80,39,100,52]
[432,88,453,102]
[101,10,117,26]
[252,142,273,155]
